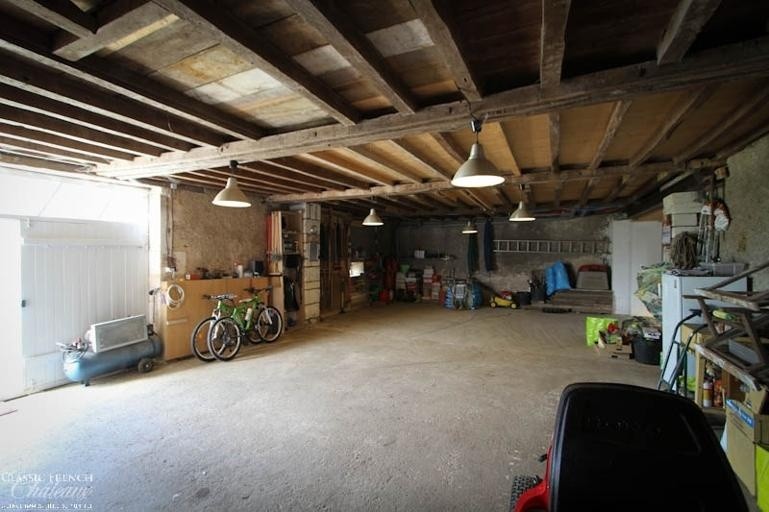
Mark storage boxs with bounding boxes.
[725,384,769,497]
[577,271,609,290]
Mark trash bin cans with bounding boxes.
[634,333,660,364]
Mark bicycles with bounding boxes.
[191,285,283,361]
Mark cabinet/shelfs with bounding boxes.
[266,210,305,335]
[320,208,351,321]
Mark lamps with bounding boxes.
[212,160,252,208]
[461,217,478,233]
[361,197,384,227]
[452,119,505,189]
[508,183,535,222]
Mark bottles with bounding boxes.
[703,379,713,408]
